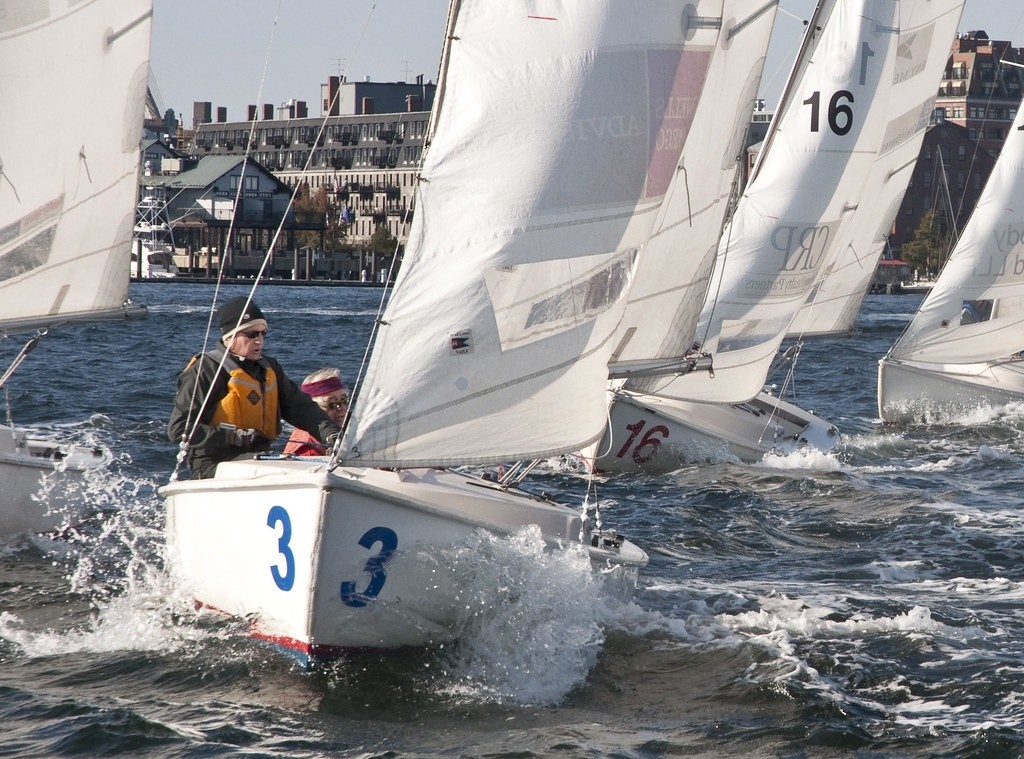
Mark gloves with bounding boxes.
[235,429,275,453]
[325,434,341,455]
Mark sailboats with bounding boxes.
[153,0,703,659]
[874,97,1022,425]
[1,0,152,537]
[530,0,969,484]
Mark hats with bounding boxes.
[216,296,269,340]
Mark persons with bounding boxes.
[284,367,354,459]
[168,297,341,481]
[959,298,992,324]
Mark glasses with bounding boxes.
[318,397,351,410]
[237,329,268,339]
